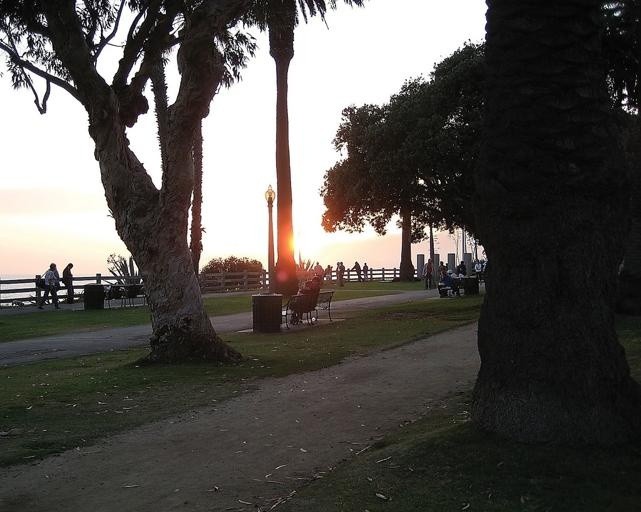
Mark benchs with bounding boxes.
[280,289,335,331]
[436,280,470,299]
[104,282,149,310]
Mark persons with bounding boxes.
[290,261,368,325]
[38,263,75,309]
[423,258,487,290]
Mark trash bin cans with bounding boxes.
[252,292,283,332]
[461,276,479,297]
[83,283,105,309]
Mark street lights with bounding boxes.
[265,185,276,294]
